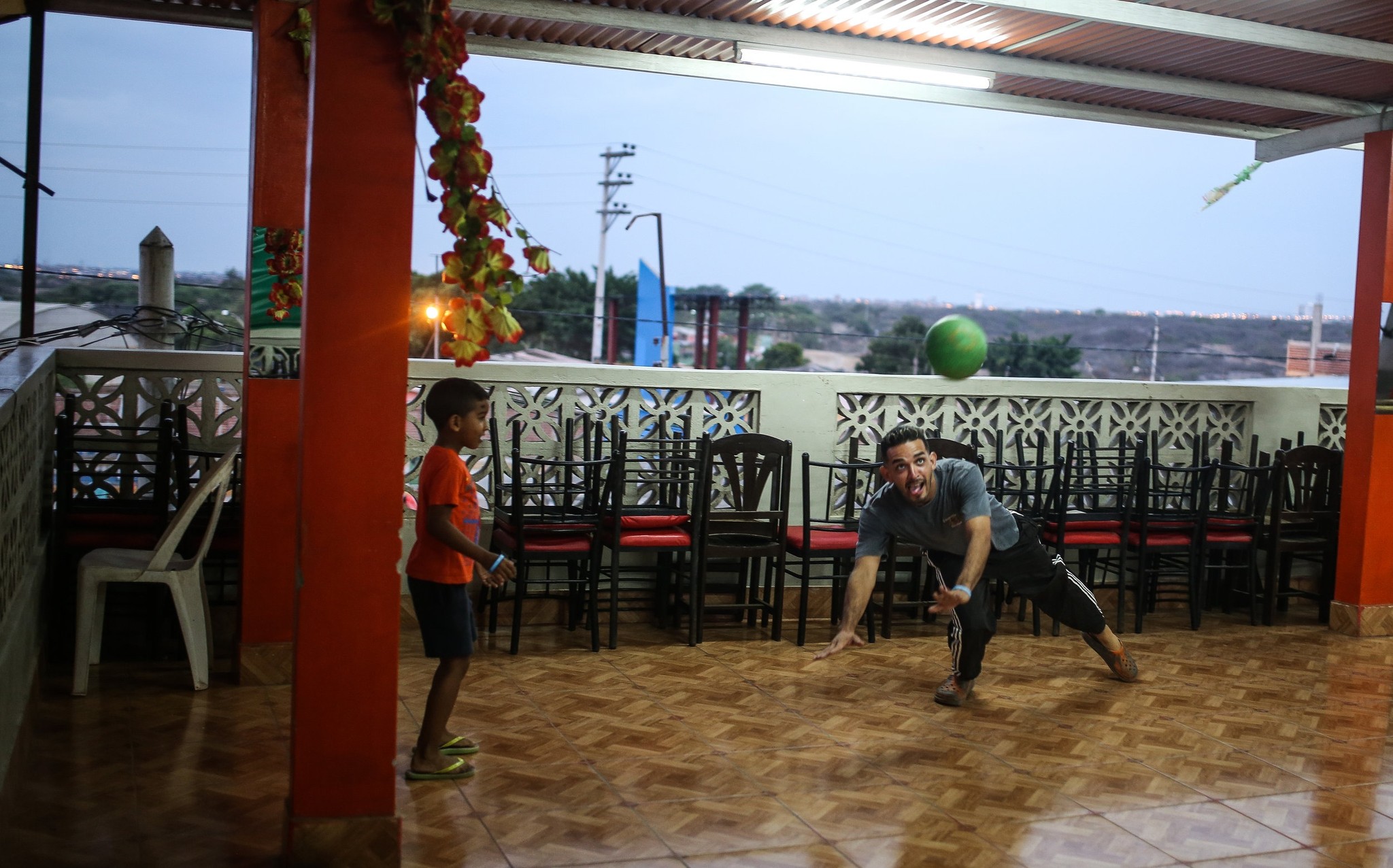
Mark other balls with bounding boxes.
[924,315,988,379]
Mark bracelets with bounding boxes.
[489,554,504,574]
[952,585,971,597]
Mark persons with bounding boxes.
[815,425,1138,704]
[405,377,516,778]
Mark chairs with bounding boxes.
[53,397,244,696]
[484,415,1344,653]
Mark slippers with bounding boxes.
[935,674,975,708]
[405,757,476,779]
[412,736,480,754]
[1081,631,1138,682]
